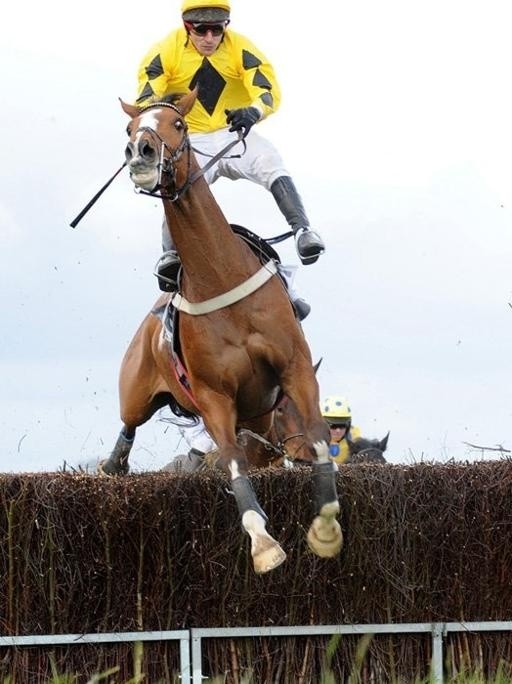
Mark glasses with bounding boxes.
[330,424,347,430]
[188,26,224,35]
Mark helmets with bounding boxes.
[182,2,233,25]
[322,401,352,425]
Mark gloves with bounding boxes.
[225,107,263,136]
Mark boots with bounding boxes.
[270,174,325,265]
[158,222,183,292]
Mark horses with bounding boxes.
[342,429,391,467]
[98,80,346,576]
[157,357,323,475]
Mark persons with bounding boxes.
[322,401,359,465]
[133,0,324,293]
